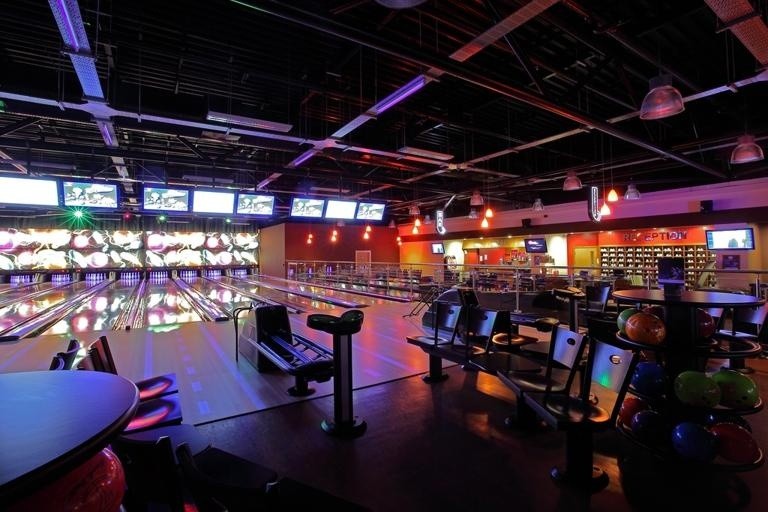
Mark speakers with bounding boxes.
[700,200,713,211]
[522,219,531,226]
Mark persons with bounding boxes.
[64,184,117,208]
[292,198,324,217]
[358,204,383,219]
[237,194,273,215]
[444,254,460,281]
[143,189,189,211]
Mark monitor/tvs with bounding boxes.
[234,192,275,217]
[60,178,119,211]
[723,256,741,271]
[587,185,600,217]
[0,177,60,207]
[357,200,387,224]
[140,183,193,214]
[324,197,359,224]
[658,257,686,286]
[705,229,755,250]
[431,243,444,254]
[190,187,238,217]
[522,238,547,254]
[288,194,327,223]
[435,210,444,228]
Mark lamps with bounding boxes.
[730,134,765,164]
[469,188,484,206]
[623,179,642,201]
[532,198,544,212]
[598,139,611,216]
[606,137,619,201]
[409,206,420,215]
[639,70,686,120]
[562,168,583,191]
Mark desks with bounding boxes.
[1,371,140,511]
[468,350,551,430]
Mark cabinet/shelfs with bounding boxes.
[435,262,569,295]
[372,262,434,304]
[597,240,716,288]
[609,287,767,510]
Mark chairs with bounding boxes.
[522,333,639,495]
[584,285,610,313]
[496,325,588,429]
[405,301,499,386]
[40,329,188,436]
